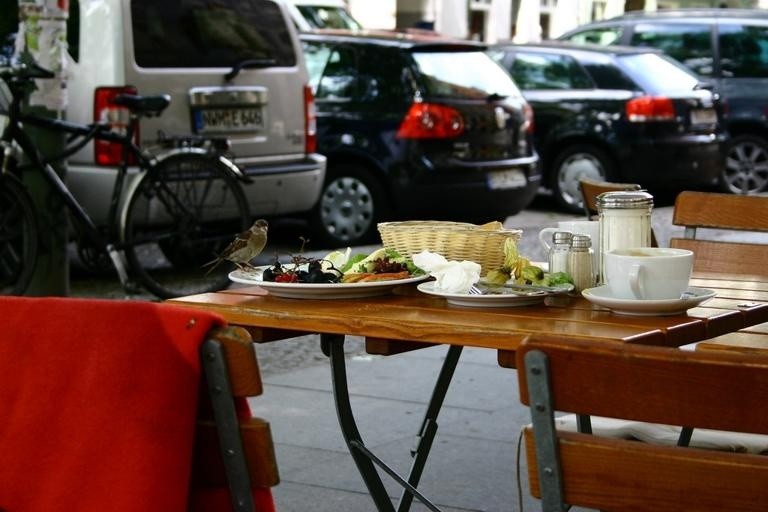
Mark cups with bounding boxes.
[606,247,694,301]
[537,220,600,274]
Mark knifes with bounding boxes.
[480,280,561,293]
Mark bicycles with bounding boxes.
[0,31,255,301]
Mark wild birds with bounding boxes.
[198,217,269,278]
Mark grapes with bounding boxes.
[263,262,336,283]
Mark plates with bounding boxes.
[418,278,575,307]
[226,263,433,298]
[583,283,717,316]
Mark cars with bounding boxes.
[481,43,733,217]
[281,2,363,33]
[296,29,542,252]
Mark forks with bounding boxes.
[468,286,540,297]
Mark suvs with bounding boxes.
[553,8,768,196]
[0,2,328,274]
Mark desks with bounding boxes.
[157,258,767,510]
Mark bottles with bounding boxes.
[568,235,596,296]
[596,190,655,287]
[550,232,574,284]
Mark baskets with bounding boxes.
[377,220,523,278]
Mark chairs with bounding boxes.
[0,292,286,512]
[665,186,768,357]
[499,326,766,510]
[575,176,661,250]
